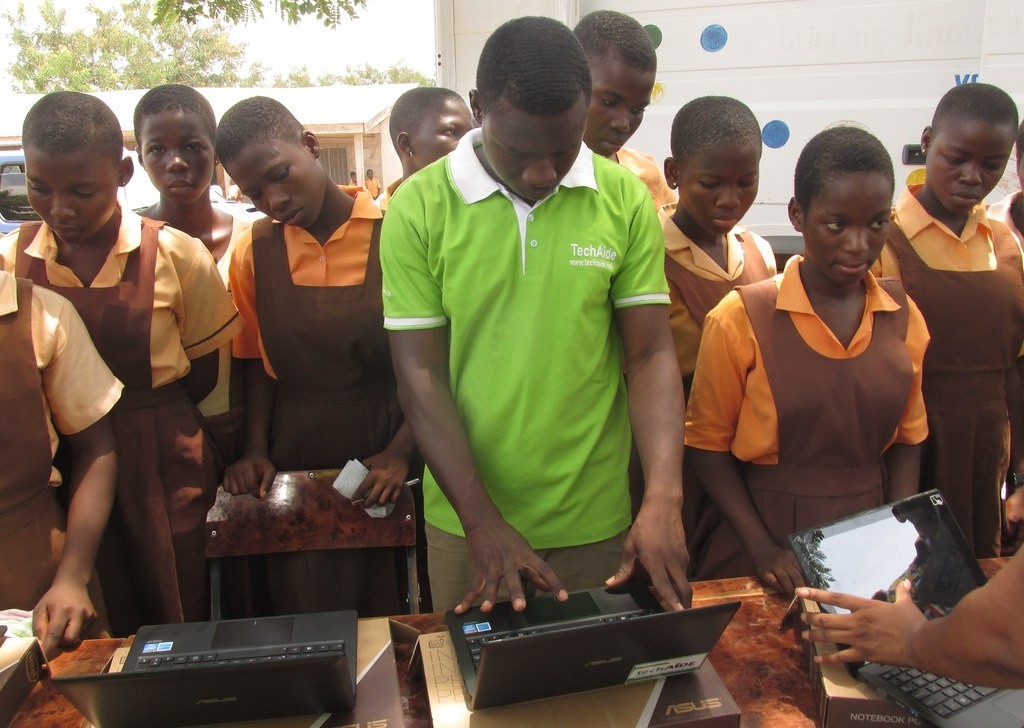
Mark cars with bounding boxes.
[0,151,45,235]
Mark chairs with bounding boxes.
[205,467,423,622]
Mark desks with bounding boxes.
[82,555,1019,728]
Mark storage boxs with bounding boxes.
[97,616,420,728]
[778,594,925,728]
[0,636,52,728]
[406,630,742,728]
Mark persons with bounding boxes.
[365,169,381,200]
[870,83,1024,558]
[130,84,251,623]
[799,545,1024,689]
[0,91,246,639]
[574,9,676,212]
[349,172,356,186]
[379,15,691,615]
[683,125,932,598]
[376,86,475,212]
[0,269,125,656]
[227,178,242,203]
[658,96,778,405]
[215,94,416,620]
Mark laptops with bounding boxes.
[444,580,741,711]
[788,488,1024,728]
[50,609,357,728]
